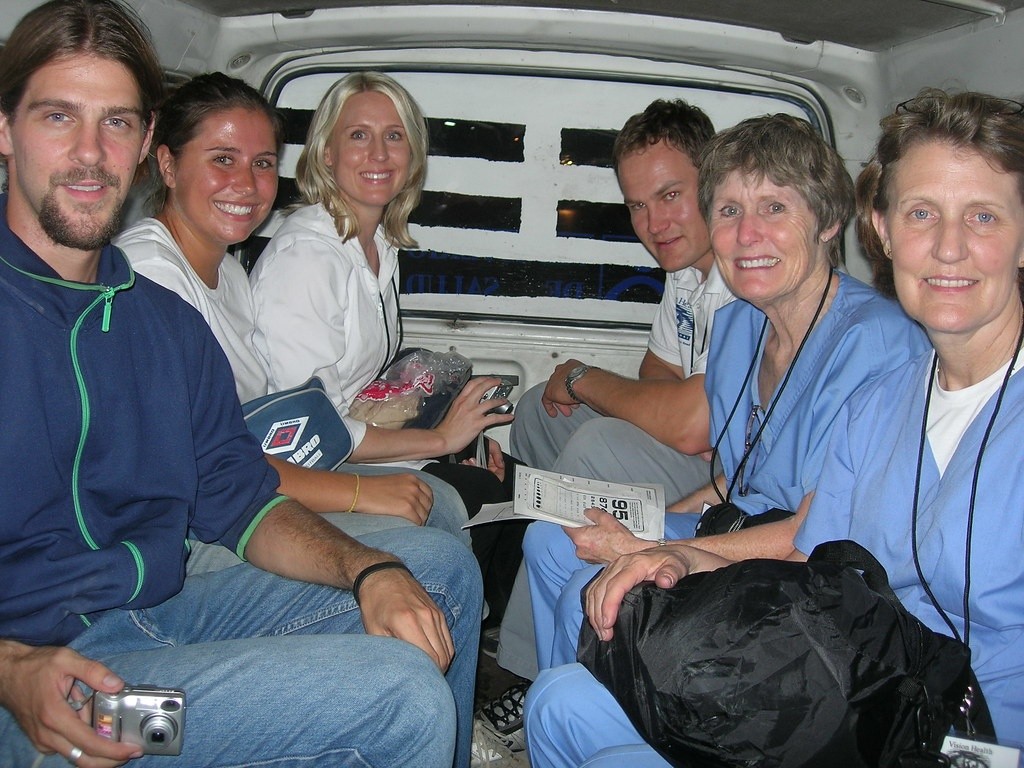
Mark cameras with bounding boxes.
[480,379,515,417]
[92,686,187,756]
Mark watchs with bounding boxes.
[565,364,601,402]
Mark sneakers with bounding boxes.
[476,685,529,751]
[467,726,528,767]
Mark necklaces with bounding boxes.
[912,328,1024,738]
[379,283,401,379]
[711,266,833,503]
[688,308,705,374]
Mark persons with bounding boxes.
[522,113,932,673]
[248,73,532,659]
[111,71,520,768]
[474,98,739,752]
[1,0,484,768]
[523,88,1024,768]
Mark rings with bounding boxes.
[68,747,83,763]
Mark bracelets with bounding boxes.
[353,562,414,605]
[659,536,666,547]
[346,471,360,513]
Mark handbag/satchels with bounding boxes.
[242,376,354,471]
[578,540,1000,767]
